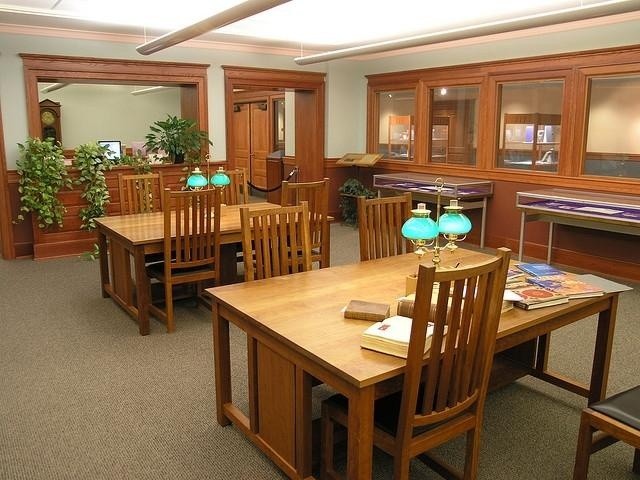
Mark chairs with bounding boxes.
[145,186,222,334]
[208,168,249,206]
[117,172,165,215]
[573,386,640,480]
[321,246,510,480]
[253,178,331,280]
[239,201,312,282]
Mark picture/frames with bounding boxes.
[98,141,122,164]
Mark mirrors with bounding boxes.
[19,53,211,170]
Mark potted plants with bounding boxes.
[143,113,214,164]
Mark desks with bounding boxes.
[515,189,640,265]
[93,203,335,336]
[205,246,622,480]
[374,172,494,249]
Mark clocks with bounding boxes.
[38,98,62,150]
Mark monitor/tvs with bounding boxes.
[98,141,121,160]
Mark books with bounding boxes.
[397,291,467,328]
[502,264,604,313]
[344,300,390,321]
[361,314,448,360]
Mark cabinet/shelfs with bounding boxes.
[500,112,561,172]
[387,114,451,164]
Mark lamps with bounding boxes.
[186,154,230,218]
[401,178,472,289]
